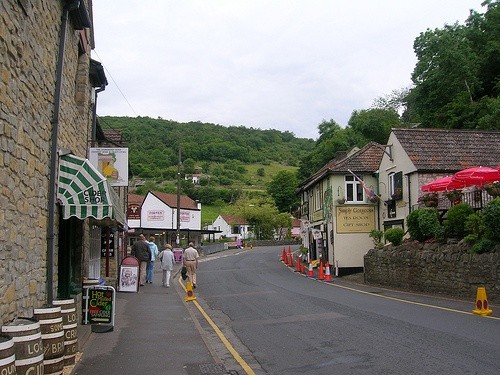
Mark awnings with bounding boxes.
[58,154,126,231]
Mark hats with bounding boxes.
[138,234,145,240]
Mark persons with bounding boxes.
[145,236,158,283]
[159,244,176,288]
[183,242,198,288]
[131,233,151,286]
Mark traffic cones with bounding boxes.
[323,261,334,282]
[294,257,301,272]
[183,281,196,302]
[300,263,306,274]
[471,287,492,316]
[287,246,291,261]
[281,247,289,264]
[306,259,316,278]
[288,255,295,268]
[316,260,325,280]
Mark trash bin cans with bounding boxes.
[172,248,184,263]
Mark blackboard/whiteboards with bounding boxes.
[85,286,115,325]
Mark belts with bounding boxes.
[186,260,194,261]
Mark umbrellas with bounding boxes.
[421,175,464,192]
[451,165,500,187]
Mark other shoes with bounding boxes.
[194,284,197,288]
[166,285,170,288]
[140,283,144,286]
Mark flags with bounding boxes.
[353,173,374,200]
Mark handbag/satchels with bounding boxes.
[160,256,163,262]
